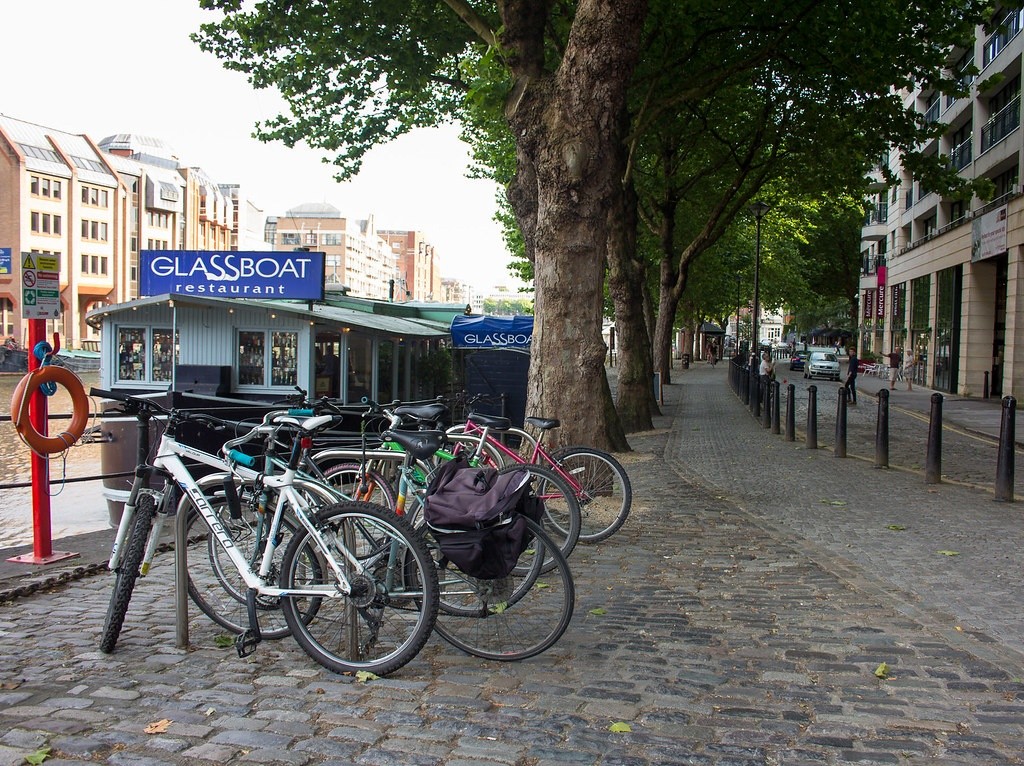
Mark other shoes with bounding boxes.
[850,401,857,404]
[847,400,851,403]
[890,388,897,390]
[906,388,912,391]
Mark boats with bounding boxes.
[0,340,102,372]
[82,281,533,524]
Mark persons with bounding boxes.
[834,341,841,355]
[768,338,796,352]
[845,347,859,404]
[315,346,339,376]
[880,347,902,390]
[709,342,718,364]
[904,349,914,391]
[760,352,775,403]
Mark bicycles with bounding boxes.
[185,386,634,661]
[711,355,715,368]
[90,387,442,676]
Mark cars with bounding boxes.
[789,351,811,370]
[803,350,841,381]
[759,339,789,352]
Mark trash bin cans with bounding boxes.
[681,353,690,369]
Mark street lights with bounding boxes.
[748,200,775,416]
[735,274,743,360]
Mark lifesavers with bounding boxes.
[9,364,90,455]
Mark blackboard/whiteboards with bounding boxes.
[652,372,663,402]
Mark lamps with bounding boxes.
[907,241,912,249]
[1013,184,1020,194]
[966,209,972,219]
[932,228,938,236]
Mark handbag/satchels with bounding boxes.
[424,457,544,580]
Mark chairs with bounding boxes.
[862,363,903,381]
